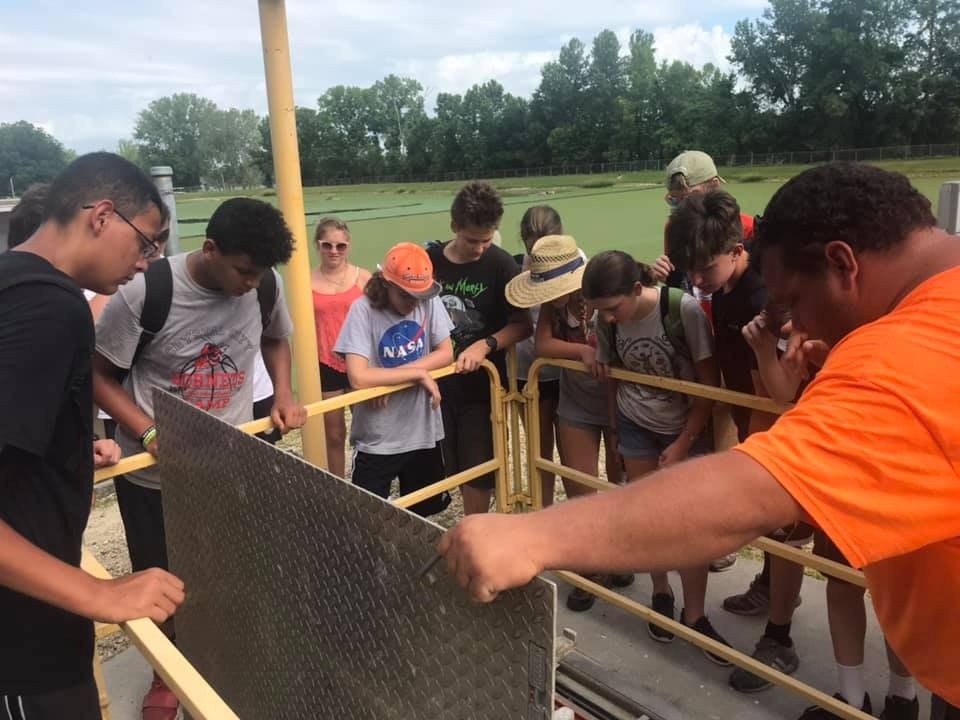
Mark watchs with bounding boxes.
[484,335,497,352]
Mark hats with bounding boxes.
[377,242,443,300]
[504,235,586,308]
[666,150,727,191]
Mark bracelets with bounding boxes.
[142,429,156,448]
[683,429,697,441]
[140,425,156,445]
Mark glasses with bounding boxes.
[82,204,159,259]
[317,239,349,253]
[665,190,700,207]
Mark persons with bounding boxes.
[309,217,373,481]
[426,150,920,720]
[433,162,960,720]
[329,242,452,518]
[0,152,307,720]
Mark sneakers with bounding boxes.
[648,584,675,641]
[680,608,735,667]
[723,573,807,614]
[728,636,799,692]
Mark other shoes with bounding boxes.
[567,574,608,611]
[795,691,872,720]
[710,553,736,571]
[879,694,920,720]
[141,679,179,720]
[606,573,635,587]
